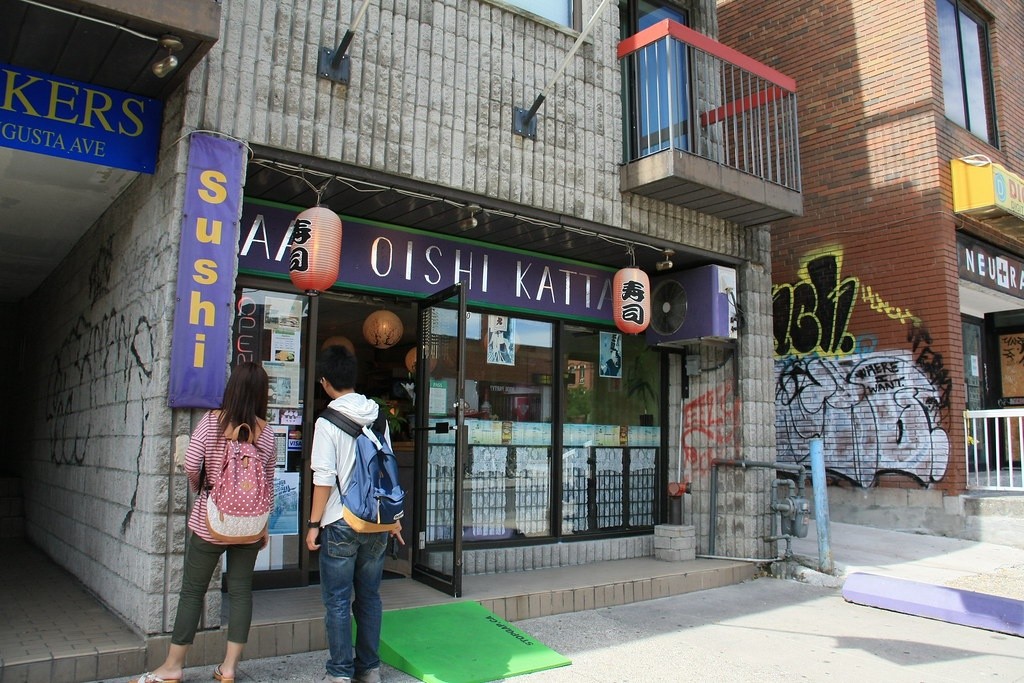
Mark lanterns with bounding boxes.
[613,266,650,336]
[290,205,343,297]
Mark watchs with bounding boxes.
[307,519,320,528]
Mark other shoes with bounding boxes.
[321,671,351,683]
[360,667,381,683]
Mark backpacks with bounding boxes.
[207,411,270,545]
[322,403,405,533]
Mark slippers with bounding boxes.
[130,672,180,683]
[213,664,234,683]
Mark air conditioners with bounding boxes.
[644,264,737,346]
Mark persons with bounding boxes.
[131,361,278,683]
[306,344,406,683]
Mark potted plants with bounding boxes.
[624,356,655,426]
[406,372,415,440]
